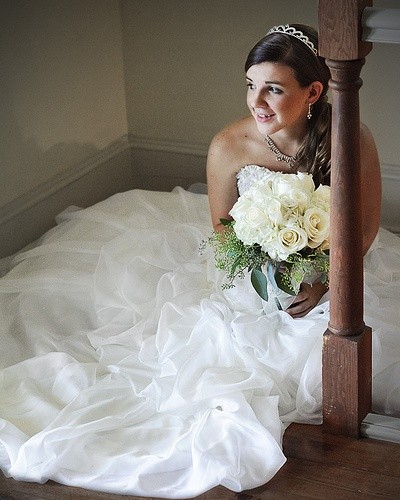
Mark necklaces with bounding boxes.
[264,134,308,169]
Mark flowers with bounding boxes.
[196,164,334,310]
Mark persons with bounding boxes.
[0,23,400,499]
